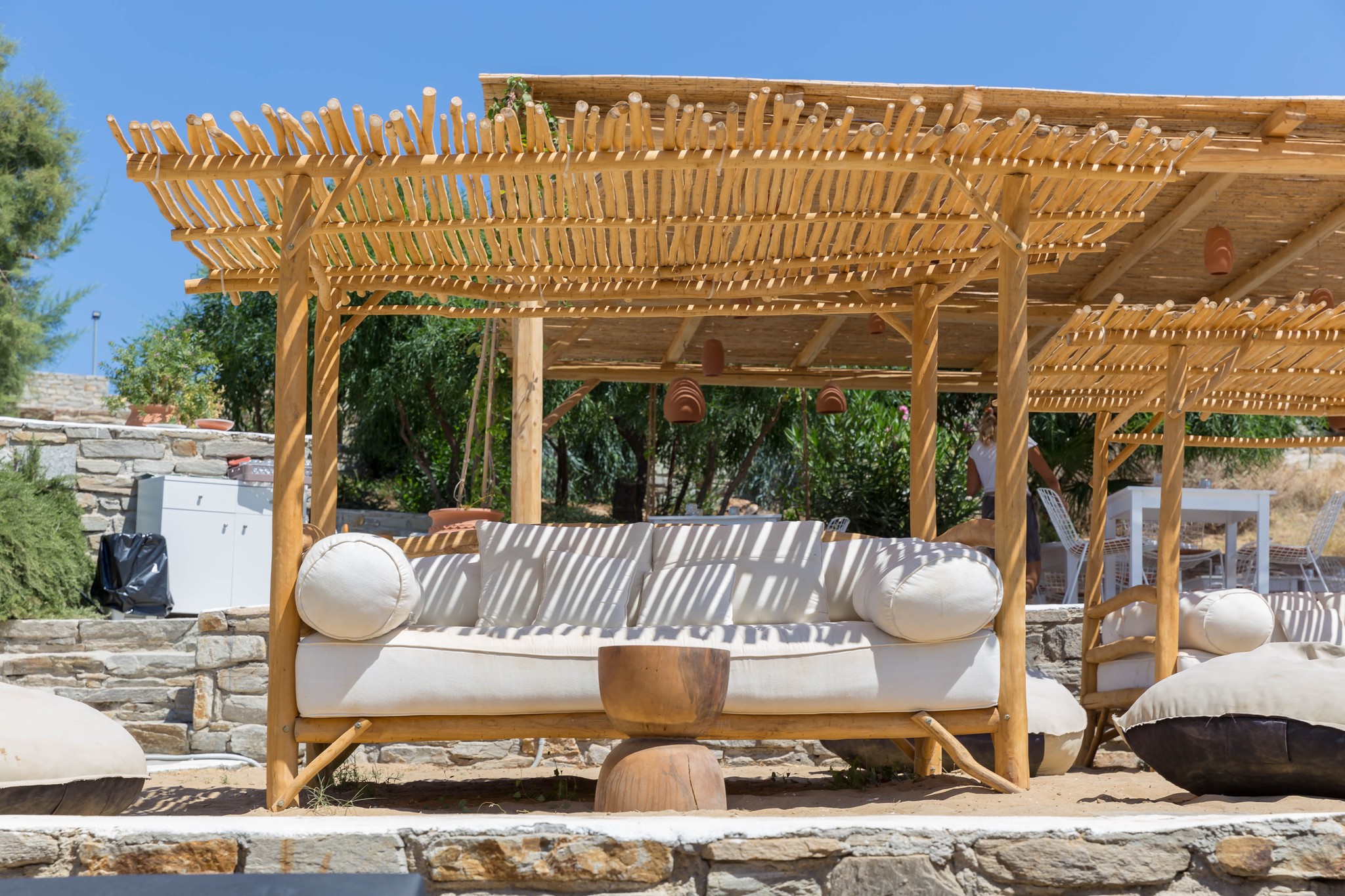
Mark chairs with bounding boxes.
[827,517,850,532]
[1036,488,1345,604]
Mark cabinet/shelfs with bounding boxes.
[136,476,308,614]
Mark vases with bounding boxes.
[428,508,505,533]
[126,405,181,426]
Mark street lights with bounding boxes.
[91,310,101,375]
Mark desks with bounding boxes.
[595,645,731,812]
[1103,486,1277,601]
[1040,541,1195,603]
[649,514,782,525]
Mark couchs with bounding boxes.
[1074,585,1345,769]
[267,519,1032,812]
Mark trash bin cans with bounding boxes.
[99,534,166,618]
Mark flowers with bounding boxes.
[96,326,229,427]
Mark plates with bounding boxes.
[192,418,234,431]
[146,424,186,428]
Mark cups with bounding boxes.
[685,503,702,516]
[1198,478,1211,488]
[729,507,740,515]
[1153,472,1162,487]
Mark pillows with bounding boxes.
[1263,591,1345,645]
[475,520,829,627]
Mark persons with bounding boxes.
[967,399,1069,603]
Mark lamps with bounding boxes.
[817,342,847,414]
[1204,195,1233,276]
[664,351,706,424]
[868,313,885,335]
[703,316,724,377]
[1308,241,1335,321]
